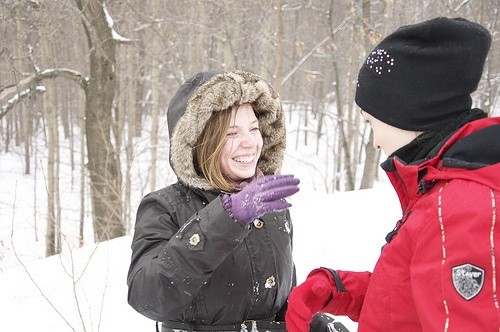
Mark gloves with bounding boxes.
[222,174,301,224]
[285,270,333,332]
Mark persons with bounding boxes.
[126,69,349,332]
[284,16,500,332]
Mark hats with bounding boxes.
[355,17,492,131]
